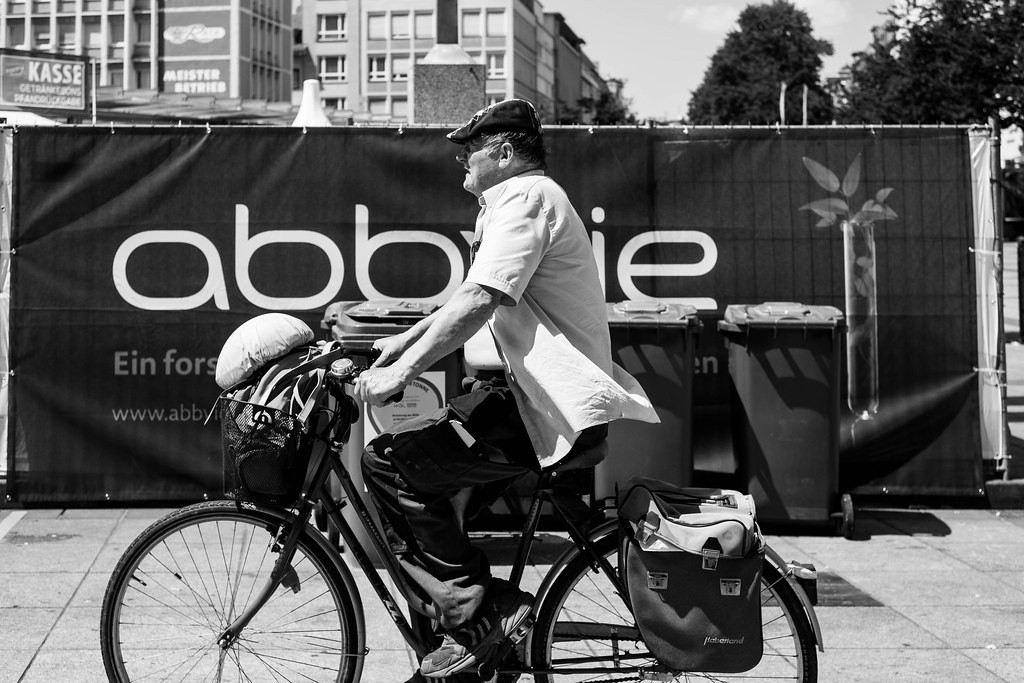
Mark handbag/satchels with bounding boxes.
[616,477,767,673]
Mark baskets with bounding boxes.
[218,394,308,508]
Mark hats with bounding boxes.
[446,99,543,145]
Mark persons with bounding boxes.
[353,98,663,683]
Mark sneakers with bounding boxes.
[421,577,536,678]
[405,669,519,683]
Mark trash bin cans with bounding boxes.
[718,302,855,540]
[592,300,718,525]
[319,300,505,570]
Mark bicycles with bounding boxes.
[99,340,824,683]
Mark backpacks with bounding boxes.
[225,338,339,503]
